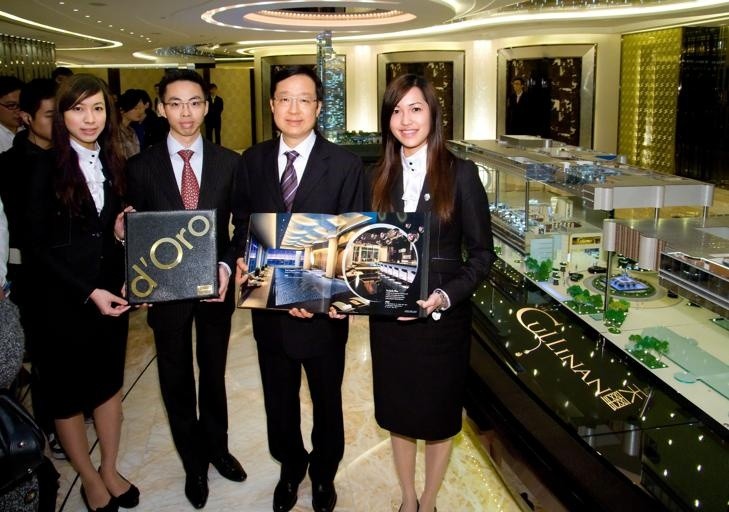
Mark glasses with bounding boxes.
[163,101,205,109]
[0,102,18,110]
[275,97,318,107]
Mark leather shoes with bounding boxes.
[209,452,247,482]
[80,479,120,512]
[273,477,300,512]
[185,468,210,508]
[310,475,337,512]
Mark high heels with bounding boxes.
[398,499,420,512]
[97,464,141,509]
[433,507,437,512]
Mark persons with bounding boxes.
[8,73,140,512]
[206,81,223,149]
[122,70,247,509]
[0,76,128,462]
[508,80,533,132]
[241,66,370,512]
[0,195,13,312]
[50,67,72,84]
[135,88,161,143]
[116,89,140,160]
[0,80,24,150]
[366,73,497,512]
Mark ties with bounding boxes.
[176,150,200,211]
[281,151,299,213]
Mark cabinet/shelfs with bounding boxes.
[334,132,383,156]
[447,140,729,512]
